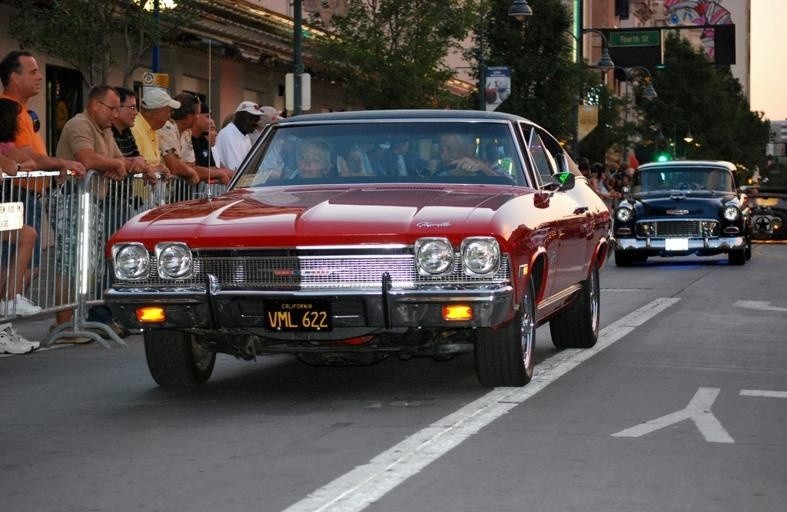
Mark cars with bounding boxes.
[609,159,754,265]
[736,183,787,241]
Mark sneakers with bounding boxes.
[0,294,43,317]
[87,320,130,339]
[1,322,41,355]
[48,325,96,344]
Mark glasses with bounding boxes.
[95,98,120,111]
[121,104,136,110]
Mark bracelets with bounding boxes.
[17,163,20,171]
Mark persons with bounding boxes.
[0,97,43,355]
[0,51,87,318]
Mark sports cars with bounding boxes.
[103,38,615,390]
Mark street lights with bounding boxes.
[573,27,613,166]
[479,1,533,110]
[621,65,658,168]
[652,118,694,160]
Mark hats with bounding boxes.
[258,105,286,127]
[236,101,265,116]
[141,87,182,110]
